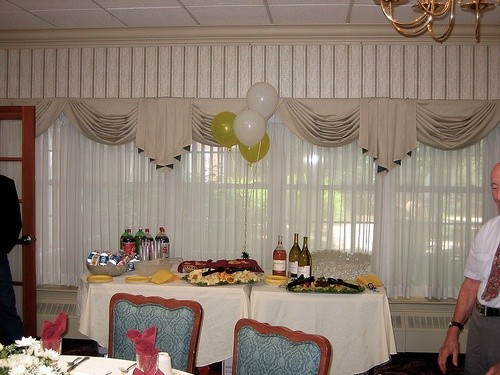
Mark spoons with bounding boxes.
[121,362,137,373]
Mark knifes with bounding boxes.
[67,357,90,372]
[68,357,81,365]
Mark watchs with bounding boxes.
[448,320,463,331]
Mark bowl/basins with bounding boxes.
[134,262,172,276]
[87,265,129,276]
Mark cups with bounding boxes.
[40,337,62,357]
[136,354,158,375]
[158,352,172,375]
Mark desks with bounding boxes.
[59,355,195,375]
[75,271,398,375]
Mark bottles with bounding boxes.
[86,250,130,266]
[121,226,170,260]
[273,233,312,278]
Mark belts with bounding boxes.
[476,301,500,317]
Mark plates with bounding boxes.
[87,274,112,282]
[264,275,288,285]
[125,276,152,285]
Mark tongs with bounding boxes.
[289,273,360,288]
[202,266,256,276]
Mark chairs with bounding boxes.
[232,316,331,375]
[107,293,203,373]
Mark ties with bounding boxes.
[481,242,500,301]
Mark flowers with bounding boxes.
[0,335,69,375]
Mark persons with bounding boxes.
[0,173,25,347]
[437,162,500,375]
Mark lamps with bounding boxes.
[374,0,500,43]
